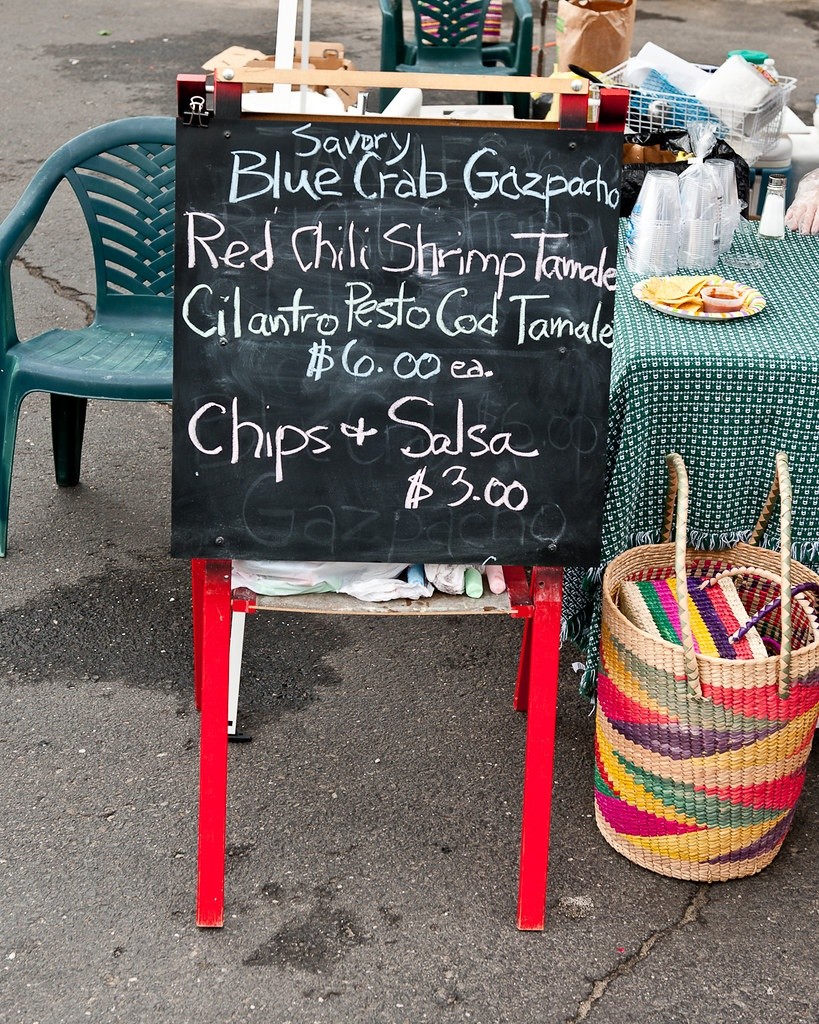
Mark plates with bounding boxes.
[632,276,766,321]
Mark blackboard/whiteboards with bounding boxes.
[171,112,625,568]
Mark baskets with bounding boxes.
[589,56,797,167]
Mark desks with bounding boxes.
[557,218,818,719]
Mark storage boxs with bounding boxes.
[201,41,365,110]
[746,135,794,219]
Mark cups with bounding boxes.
[633,160,740,274]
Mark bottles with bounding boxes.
[762,58,779,81]
[758,174,786,240]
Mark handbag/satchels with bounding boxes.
[592,453,819,886]
[555,1,636,77]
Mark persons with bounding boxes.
[784,167,819,235]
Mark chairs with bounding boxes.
[0,117,176,559]
[378,0,533,119]
[191,67,589,932]
